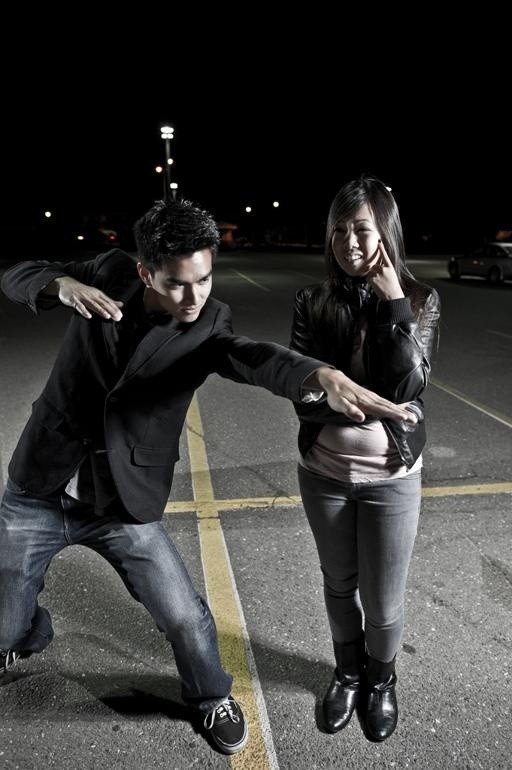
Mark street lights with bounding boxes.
[155,124,179,202]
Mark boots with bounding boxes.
[322,629,366,732]
[362,653,398,743]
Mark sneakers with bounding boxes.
[201,694,248,754]
[0,650,24,673]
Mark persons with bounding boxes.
[279,177,440,742]
[1,194,419,753]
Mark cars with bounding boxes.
[447,243,511,286]
[98,228,117,242]
